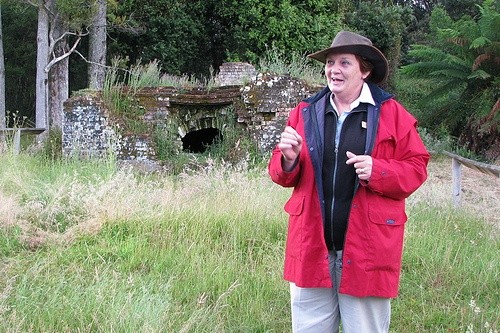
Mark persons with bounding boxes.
[267,30,429,333]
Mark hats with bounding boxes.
[307,31,389,84]
